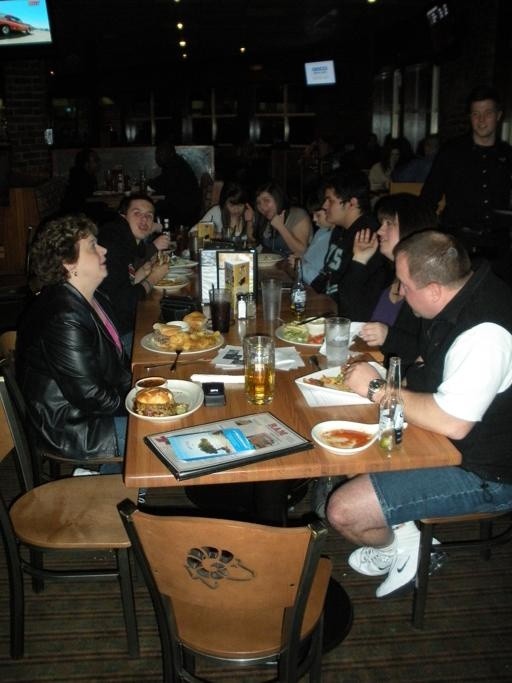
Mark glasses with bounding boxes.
[470,110,497,119]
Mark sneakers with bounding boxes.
[348,546,400,577]
[377,534,445,601]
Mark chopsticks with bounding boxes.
[297,313,339,326]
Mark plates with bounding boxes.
[141,329,224,355]
[167,260,200,269]
[276,321,343,347]
[150,275,190,293]
[126,380,205,424]
[255,254,284,267]
[312,421,408,456]
[295,362,396,406]
[165,269,194,276]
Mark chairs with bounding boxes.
[117,499,353,682]
[199,172,213,213]
[1,329,125,484]
[412,511,512,633]
[0,378,176,660]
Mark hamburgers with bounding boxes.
[152,323,177,348]
[132,388,177,416]
[183,311,209,331]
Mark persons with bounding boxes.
[415,88,512,289]
[149,141,201,233]
[15,210,132,462]
[188,179,255,239]
[318,134,335,154]
[60,148,119,228]
[303,192,336,295]
[326,229,512,598]
[23,176,138,335]
[368,142,403,191]
[424,136,439,159]
[95,193,170,316]
[322,166,395,323]
[133,235,171,272]
[255,181,314,258]
[339,192,441,385]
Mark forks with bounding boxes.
[171,348,182,371]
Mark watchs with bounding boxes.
[367,379,387,402]
[143,277,153,294]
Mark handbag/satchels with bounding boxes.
[159,289,203,326]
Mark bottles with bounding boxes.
[378,358,403,456]
[163,217,171,240]
[290,258,308,319]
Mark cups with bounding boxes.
[305,316,324,335]
[243,336,276,405]
[326,318,351,365]
[210,289,233,334]
[261,280,282,321]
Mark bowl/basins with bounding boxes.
[136,377,168,392]
